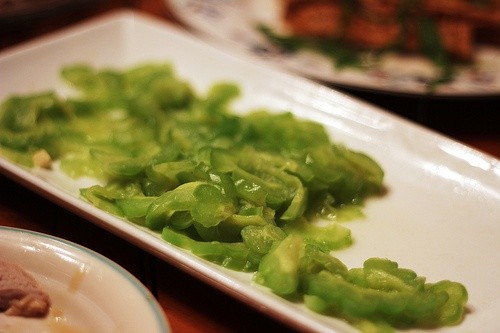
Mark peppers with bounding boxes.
[0,62,469,333]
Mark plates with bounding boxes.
[165,0,500,105]
[0,11,500,333]
[1,223,171,333]
